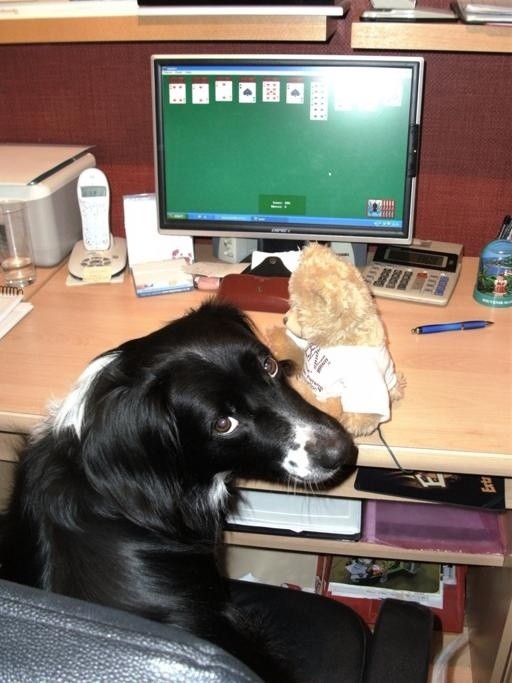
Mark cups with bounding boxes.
[473,239,512,307]
[0,200,37,288]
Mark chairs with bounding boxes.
[2,582,274,683]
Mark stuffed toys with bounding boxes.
[269,235,406,438]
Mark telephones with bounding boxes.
[67,168,128,280]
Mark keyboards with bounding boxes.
[227,488,364,540]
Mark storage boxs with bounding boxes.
[1,142,98,268]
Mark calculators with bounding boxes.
[362,238,464,306]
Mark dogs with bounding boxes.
[0,299,356,683]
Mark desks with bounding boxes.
[2,235,512,683]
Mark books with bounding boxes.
[0,299,34,339]
[450,0,511,24]
[0,284,25,321]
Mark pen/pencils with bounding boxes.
[411,321,495,335]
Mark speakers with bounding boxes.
[213,237,258,264]
[331,241,368,268]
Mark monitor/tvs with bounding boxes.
[150,54,424,276]
